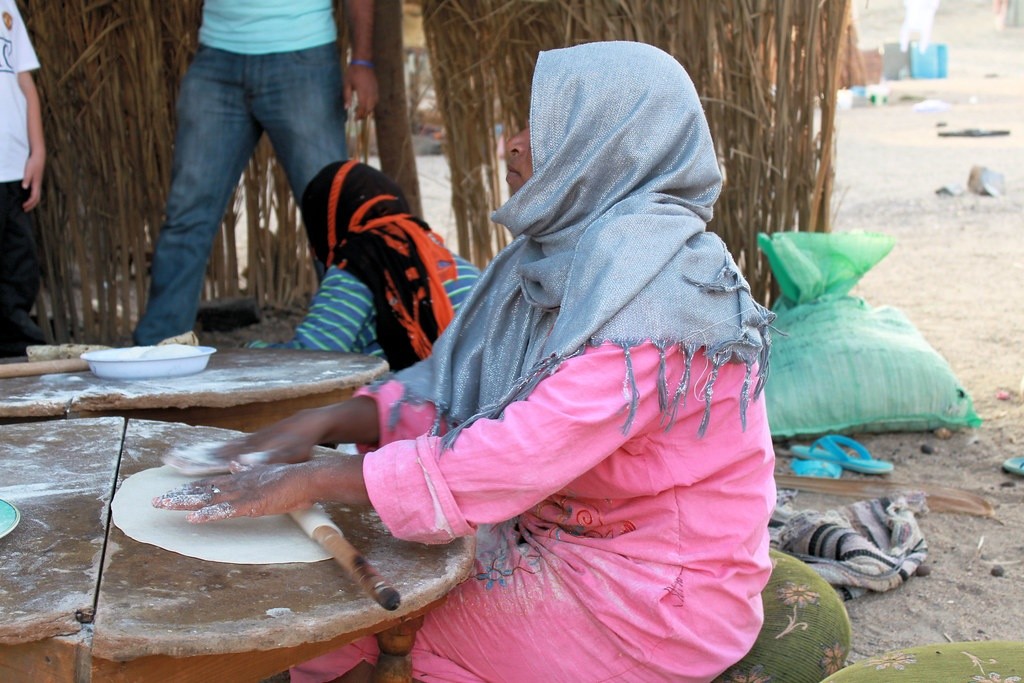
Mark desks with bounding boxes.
[0,352,392,440]
[1,417,479,683]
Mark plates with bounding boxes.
[79,345,218,379]
[0,498,21,538]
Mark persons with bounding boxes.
[130,0,379,346]
[240,158,483,372]
[150,39,789,682]
[0,0,47,359]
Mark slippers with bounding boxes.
[789,435,893,479]
[1003,457,1024,475]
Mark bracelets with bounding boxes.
[348,58,378,70]
